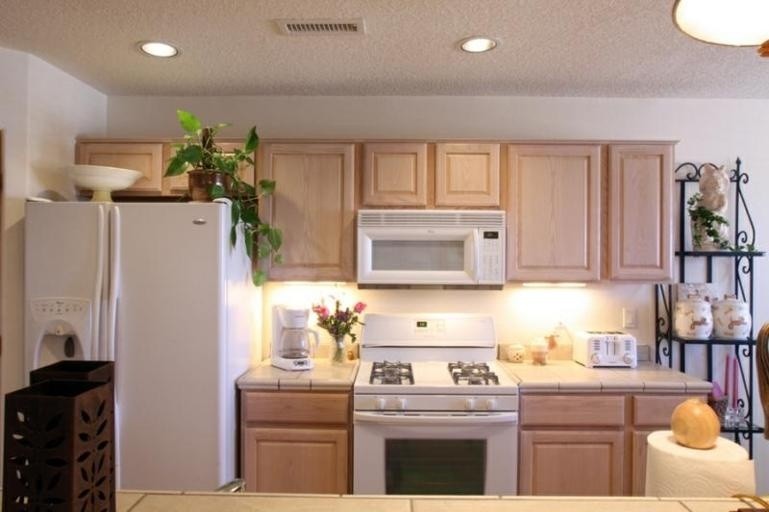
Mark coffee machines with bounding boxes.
[270,302,319,371]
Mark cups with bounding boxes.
[724,407,746,427]
[531,343,548,365]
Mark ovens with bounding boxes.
[352,411,520,495]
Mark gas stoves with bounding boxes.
[353,359,520,413]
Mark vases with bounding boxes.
[326,335,350,367]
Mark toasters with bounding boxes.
[571,329,639,368]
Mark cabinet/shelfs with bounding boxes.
[505,142,603,284]
[359,140,432,208]
[169,135,253,200]
[522,388,629,497]
[74,136,166,198]
[238,358,349,491]
[651,159,762,462]
[632,392,705,497]
[603,142,675,280]
[258,142,359,283]
[434,141,501,210]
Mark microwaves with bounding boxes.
[355,208,507,286]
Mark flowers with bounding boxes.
[312,295,367,341]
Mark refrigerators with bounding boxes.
[20,197,263,488]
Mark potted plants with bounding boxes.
[686,195,751,252]
[162,108,288,289]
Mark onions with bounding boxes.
[671,398,720,449]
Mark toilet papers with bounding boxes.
[646,431,755,496]
